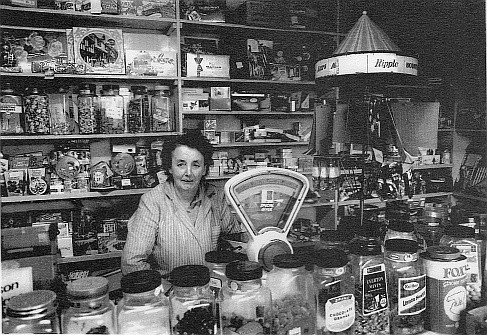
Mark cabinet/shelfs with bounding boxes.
[0,0,487,285]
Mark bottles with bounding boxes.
[1,83,174,132]
[4,208,487,335]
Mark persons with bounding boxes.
[120,132,243,277]
